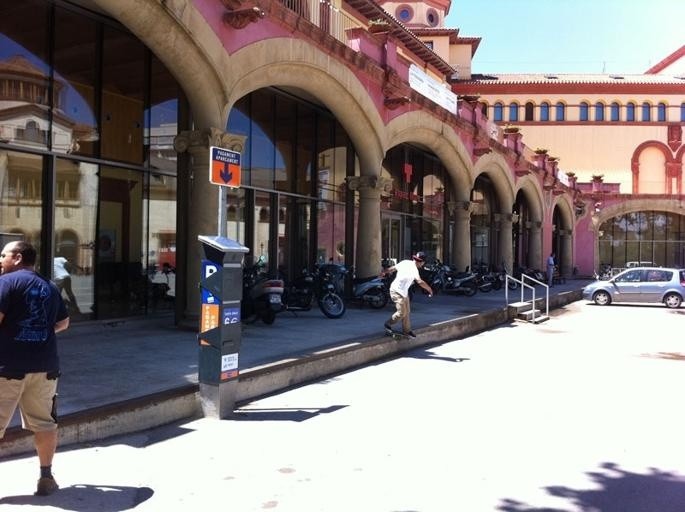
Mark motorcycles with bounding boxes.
[279,256,348,318]
[336,257,547,311]
[242,253,285,327]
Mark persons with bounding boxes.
[377,252,434,337]
[546,252,556,287]
[54,257,78,312]
[0,241,71,496]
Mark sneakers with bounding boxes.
[35,476,60,496]
[402,330,417,340]
[383,322,394,335]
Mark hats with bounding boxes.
[411,252,425,264]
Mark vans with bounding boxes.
[624,261,661,269]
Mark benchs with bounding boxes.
[553,267,565,284]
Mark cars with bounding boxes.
[580,265,685,308]
